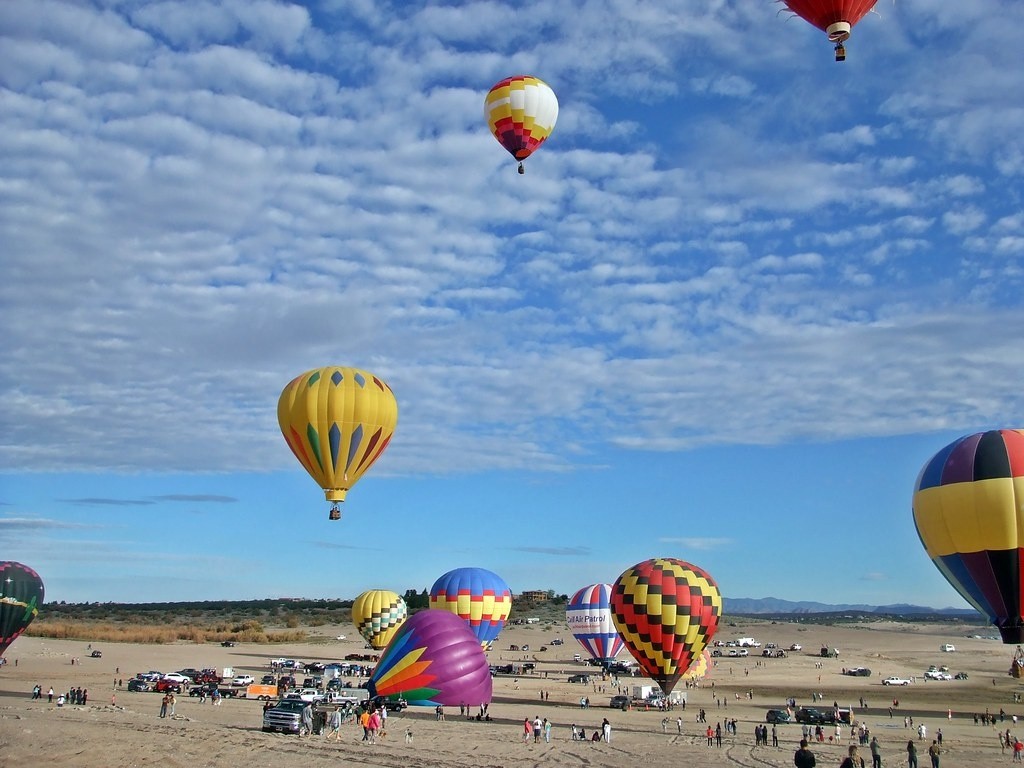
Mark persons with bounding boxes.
[813,661,823,668]
[263,701,276,715]
[658,659,779,747]
[31,683,88,706]
[785,692,951,768]
[910,676,927,683]
[200,688,221,706]
[159,694,176,718]
[974,678,1024,768]
[512,654,628,743]
[72,657,79,665]
[114,679,122,690]
[298,696,493,744]
[112,695,115,705]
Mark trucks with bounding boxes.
[222,668,235,678]
[313,692,358,706]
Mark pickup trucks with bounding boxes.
[189,684,238,699]
[137,670,166,681]
[221,642,235,647]
[263,699,328,735]
[882,677,911,686]
[288,688,324,706]
[232,674,255,686]
[362,696,402,712]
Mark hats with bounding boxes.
[59,694,65,696]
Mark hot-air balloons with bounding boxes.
[484,75,559,175]
[913,428,1023,682]
[679,649,711,681]
[277,365,399,520]
[0,560,45,664]
[365,610,493,707]
[608,557,723,709]
[780,0,877,61]
[565,584,626,680]
[428,567,512,651]
[351,589,407,662]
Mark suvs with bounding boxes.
[852,668,871,677]
[568,674,589,683]
[848,667,863,673]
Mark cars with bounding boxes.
[365,643,372,649]
[92,650,102,657]
[260,676,277,685]
[278,676,297,688]
[271,658,361,671]
[175,669,201,677]
[574,654,631,673]
[159,673,191,684]
[609,695,631,708]
[821,710,850,726]
[794,708,823,725]
[551,640,563,645]
[303,676,342,689]
[924,665,968,681]
[337,635,347,640]
[487,634,547,652]
[711,638,802,658]
[766,709,791,725]
[128,680,149,691]
[194,674,224,685]
[488,664,536,677]
[644,694,663,708]
